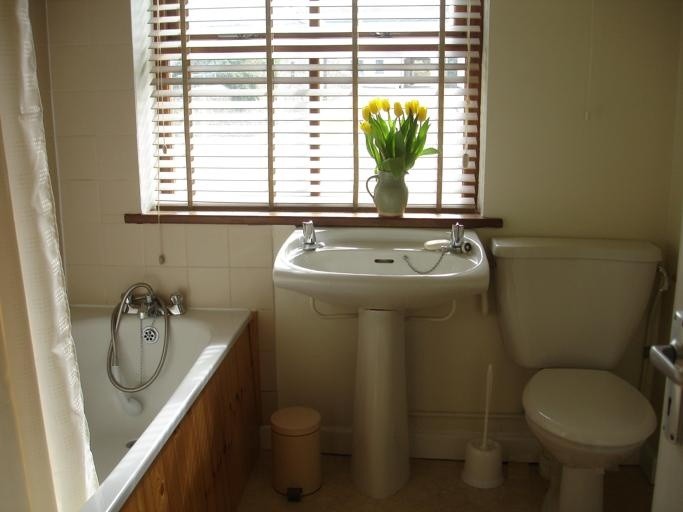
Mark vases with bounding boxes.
[365,170,409,218]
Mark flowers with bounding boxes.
[359,97,440,173]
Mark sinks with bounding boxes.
[272,228,491,307]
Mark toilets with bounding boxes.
[492,237,663,512]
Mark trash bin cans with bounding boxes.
[270,406,322,502]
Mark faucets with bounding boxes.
[303,221,324,251]
[135,303,152,320]
[450,223,472,253]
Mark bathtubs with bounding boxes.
[70,305,259,512]
